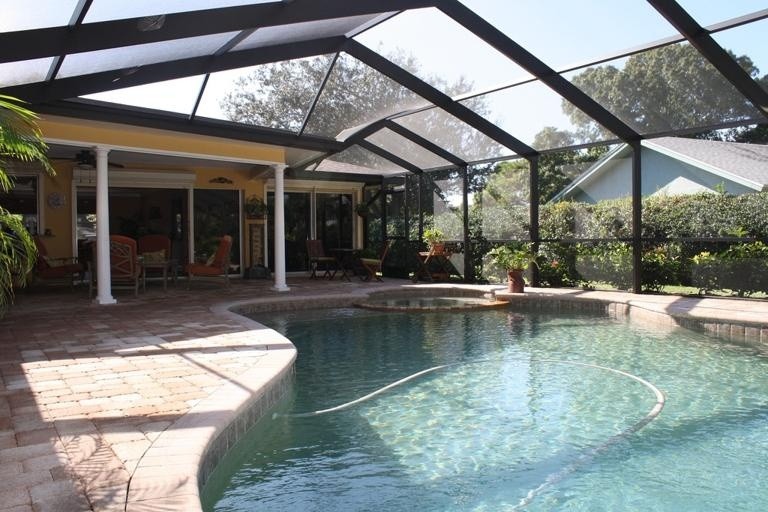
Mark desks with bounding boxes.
[141,260,171,292]
[327,246,364,284]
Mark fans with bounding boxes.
[47,149,123,169]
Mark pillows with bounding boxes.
[42,254,64,268]
[141,249,168,265]
[206,252,216,267]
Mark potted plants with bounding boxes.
[483,239,536,294]
[421,228,446,255]
[352,202,370,218]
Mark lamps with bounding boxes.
[77,159,95,183]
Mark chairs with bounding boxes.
[184,234,233,291]
[87,234,146,300]
[28,234,86,294]
[135,233,178,285]
[358,241,388,283]
[302,237,337,283]
